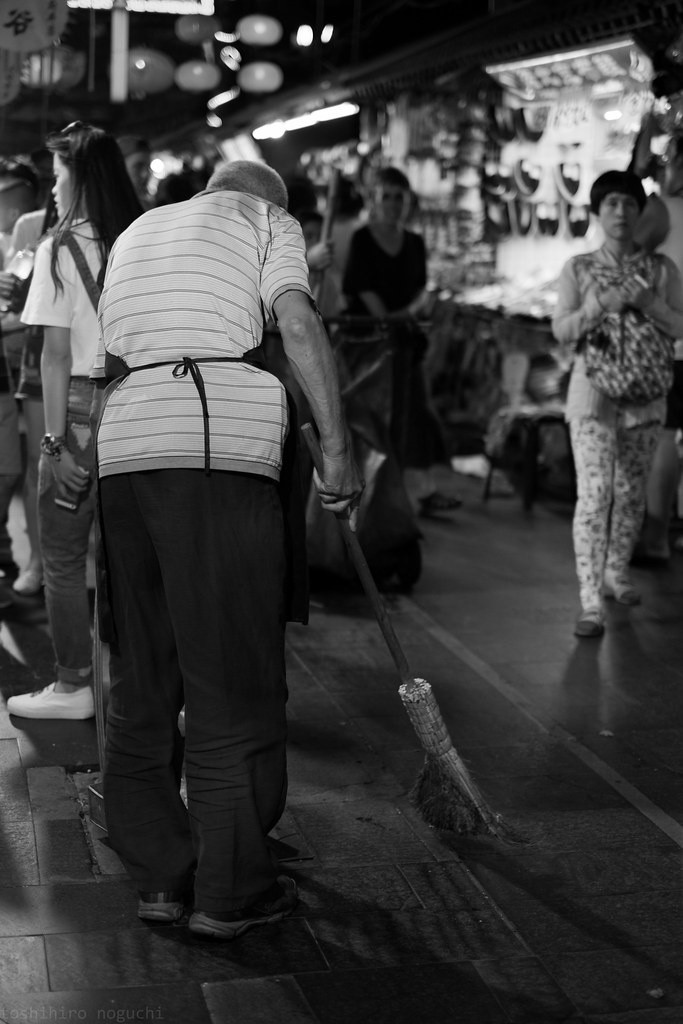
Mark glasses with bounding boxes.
[61,121,84,164]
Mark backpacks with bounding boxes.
[575,251,672,404]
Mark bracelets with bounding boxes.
[40,434,66,455]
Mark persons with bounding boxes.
[154,168,462,511]
[555,170,683,635]
[0,123,145,719]
[635,127,683,551]
[94,160,366,936]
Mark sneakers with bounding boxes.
[134,879,188,921]
[6,680,95,720]
[190,876,297,937]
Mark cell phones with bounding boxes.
[54,466,84,510]
[635,275,649,290]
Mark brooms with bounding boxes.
[298,421,536,847]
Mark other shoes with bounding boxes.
[419,490,463,510]
[12,561,44,592]
[628,542,673,569]
[573,607,608,637]
[602,578,640,604]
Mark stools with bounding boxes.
[481,417,578,511]
